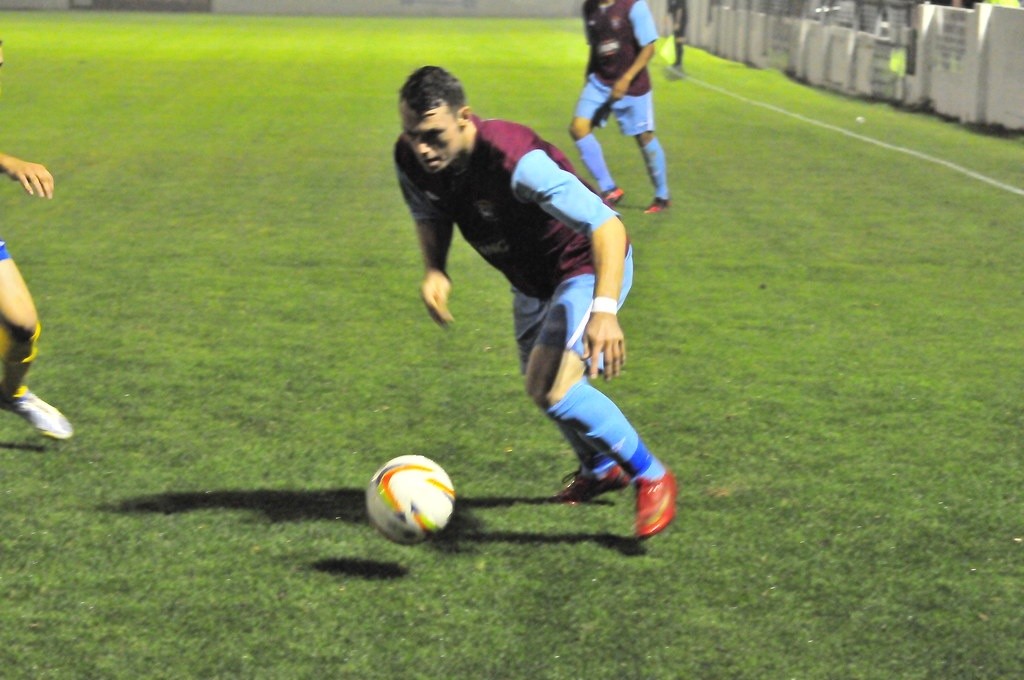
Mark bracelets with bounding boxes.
[591,297,617,314]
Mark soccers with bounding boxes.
[364,454,455,546]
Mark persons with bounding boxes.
[931,0,984,10]
[668,0,688,67]
[0,41,75,440]
[569,0,672,213]
[393,67,676,539]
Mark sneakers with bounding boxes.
[636,469,677,541]
[599,187,624,206]
[550,463,632,503]
[644,196,672,214]
[0,385,74,440]
